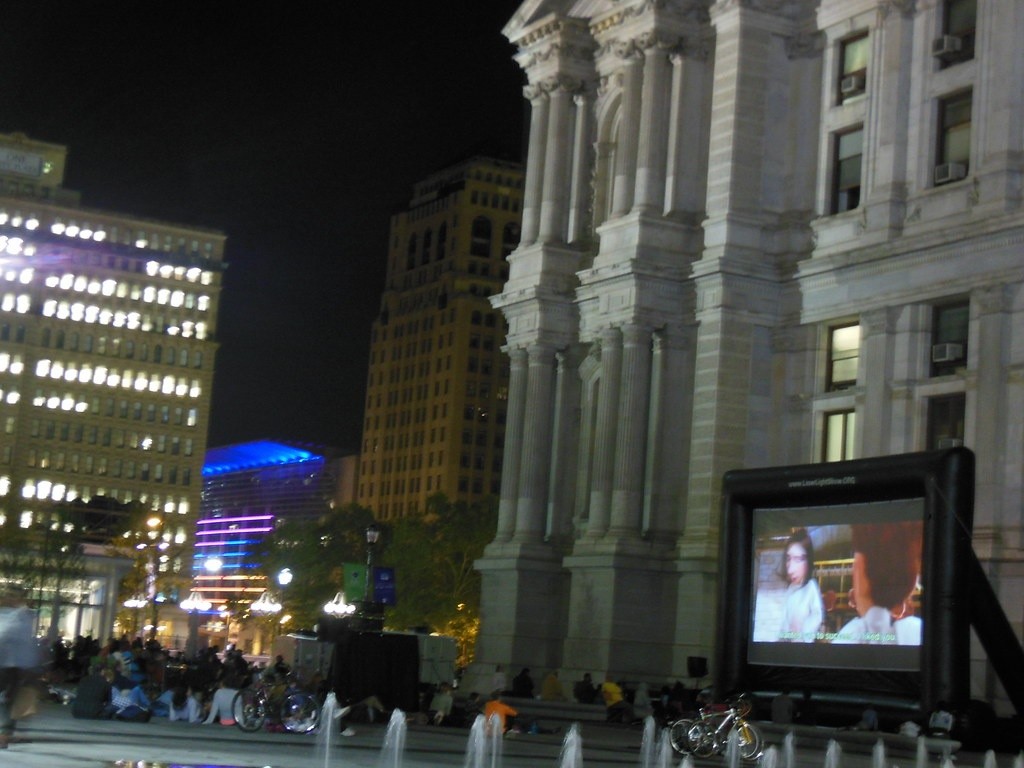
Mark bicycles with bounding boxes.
[668,690,766,762]
[230,667,322,735]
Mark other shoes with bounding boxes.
[0,736,8,748]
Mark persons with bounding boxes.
[0,607,356,748]
[512,668,535,697]
[849,703,879,732]
[461,691,482,728]
[632,681,654,717]
[653,682,694,729]
[544,666,567,704]
[793,689,816,726]
[602,676,639,725]
[420,681,453,728]
[484,691,517,740]
[494,665,510,697]
[572,673,598,703]
[771,687,792,723]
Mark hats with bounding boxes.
[469,693,479,700]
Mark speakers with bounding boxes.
[688,656,709,678]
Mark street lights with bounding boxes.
[276,566,295,640]
[362,524,381,601]
